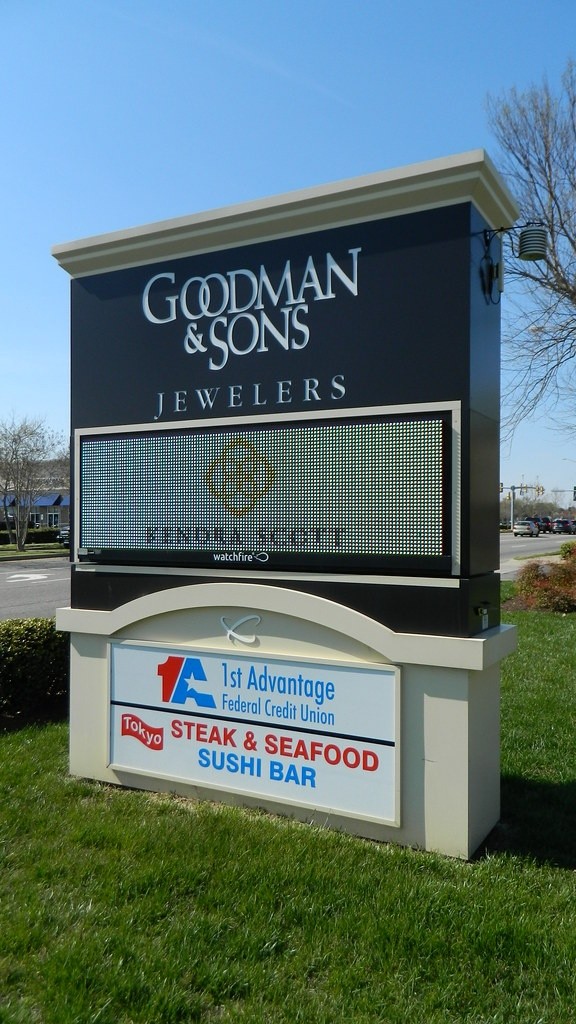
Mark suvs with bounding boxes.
[521,516,576,535]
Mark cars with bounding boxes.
[513,521,540,537]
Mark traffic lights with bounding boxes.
[540,486,545,495]
[537,487,539,497]
